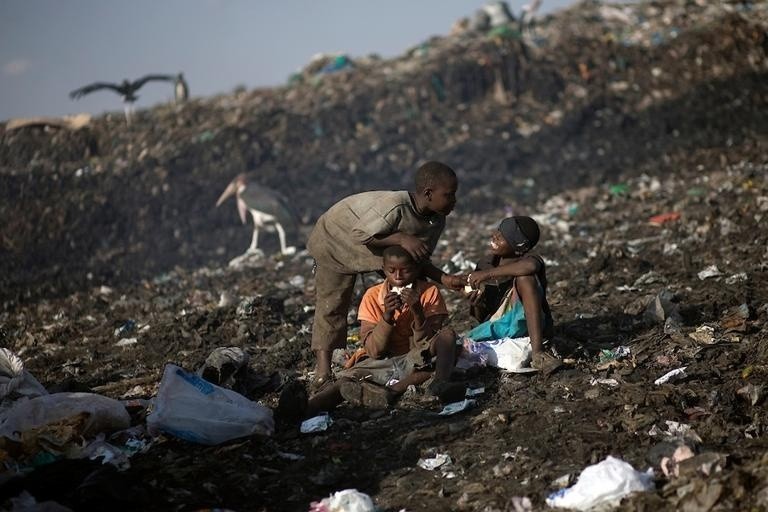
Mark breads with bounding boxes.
[464,274,486,295]
[390,283,414,296]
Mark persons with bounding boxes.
[306,162,470,393]
[463,216,563,375]
[337,246,467,410]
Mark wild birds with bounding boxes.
[215,172,298,256]
[174,70,190,104]
[68,74,174,103]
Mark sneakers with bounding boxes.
[428,380,466,402]
[530,351,563,374]
[274,380,308,431]
[339,381,395,410]
[310,374,338,392]
